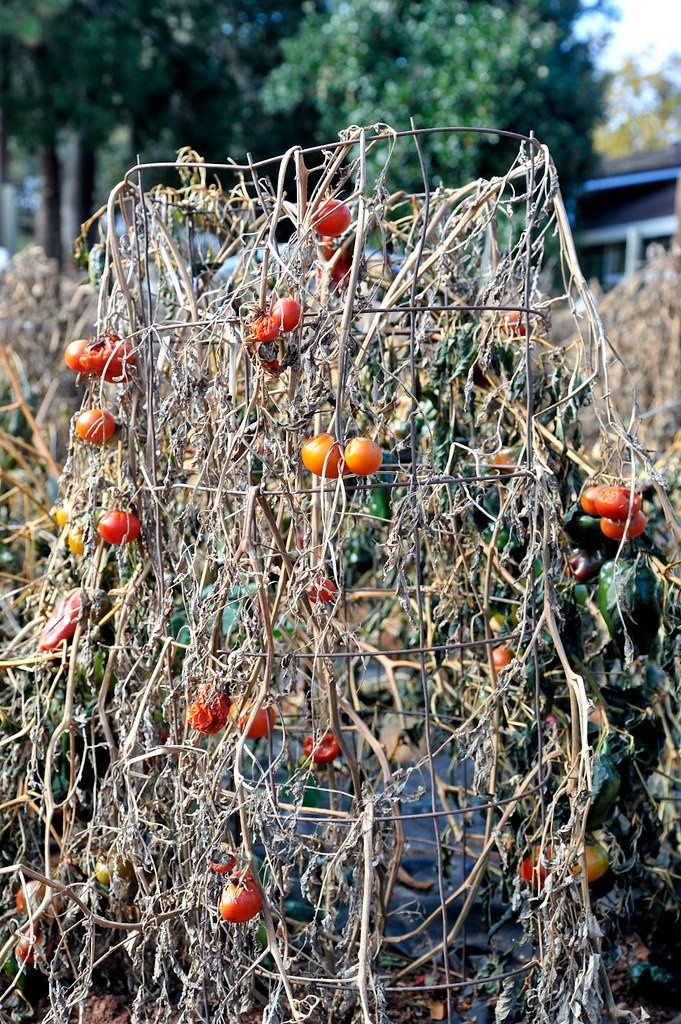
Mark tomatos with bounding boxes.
[16,197,655,967]
